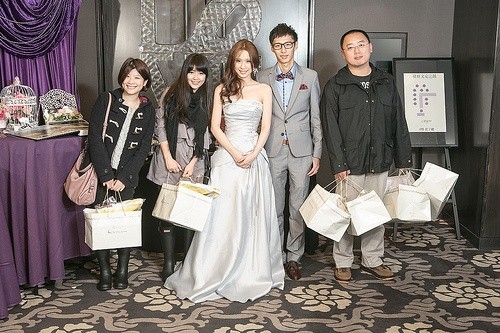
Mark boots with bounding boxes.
[160,234,174,282]
[95,250,113,290]
[114,249,130,290]
[184,228,194,264]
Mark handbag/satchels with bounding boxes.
[63,149,98,206]
[299,177,352,242]
[383,168,431,223]
[169,175,220,232]
[403,161,459,219]
[342,176,392,236]
[152,171,177,224]
[82,186,143,250]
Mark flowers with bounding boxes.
[0,93,78,120]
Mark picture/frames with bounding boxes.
[366,32,458,147]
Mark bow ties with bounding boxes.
[276,72,295,81]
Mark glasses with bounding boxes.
[342,42,369,49]
[272,40,296,50]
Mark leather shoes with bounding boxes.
[285,260,301,281]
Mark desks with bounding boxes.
[0,128,95,319]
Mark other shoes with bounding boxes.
[360,264,394,280]
[334,267,351,281]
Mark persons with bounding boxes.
[163,39,286,303]
[255,23,324,279]
[146,53,214,282]
[319,30,412,280]
[79,58,156,290]
